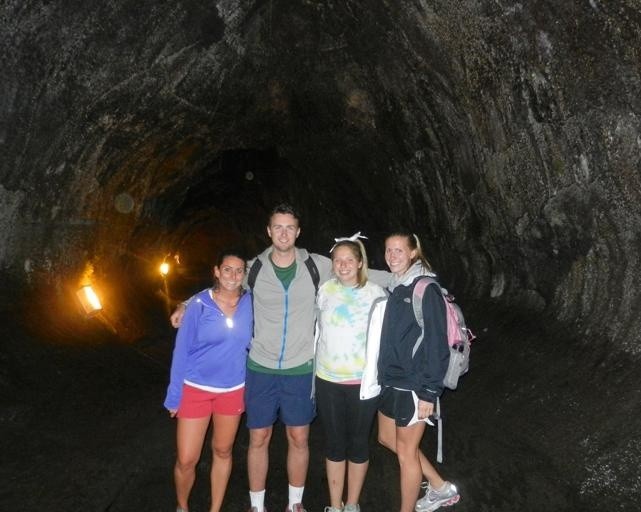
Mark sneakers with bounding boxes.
[250,503,360,512]
[414,481,460,512]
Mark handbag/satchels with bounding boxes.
[412,277,470,390]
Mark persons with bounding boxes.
[376,228,460,511]
[310,230,391,511]
[169,203,399,511]
[162,244,256,511]
